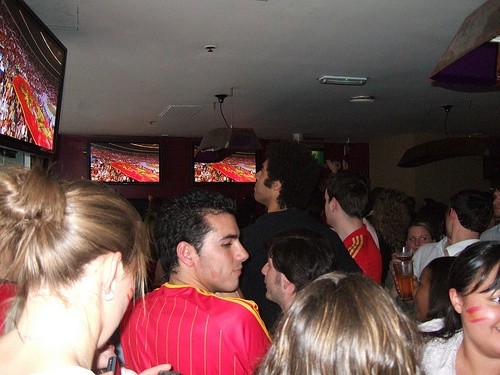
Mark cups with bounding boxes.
[396,247,412,262]
[394,261,413,300]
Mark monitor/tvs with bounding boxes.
[88,141,162,185]
[0,0,68,160]
[190,143,259,184]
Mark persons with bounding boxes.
[0,139,500,375]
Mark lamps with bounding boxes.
[319,75,367,85]
[194,94,261,163]
